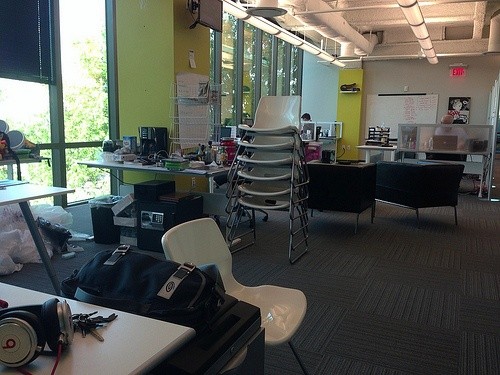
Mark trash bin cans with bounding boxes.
[91,194,126,244]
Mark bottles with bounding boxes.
[198,140,228,168]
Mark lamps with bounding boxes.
[223,0,438,63]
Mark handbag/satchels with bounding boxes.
[174,195,203,225]
[61,245,226,325]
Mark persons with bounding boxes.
[301,112,312,122]
[435,114,466,148]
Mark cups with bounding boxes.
[322,150,331,163]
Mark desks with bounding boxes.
[0,281,196,375]
[356,145,396,164]
[0,180,77,297]
[397,123,493,196]
[77,156,243,225]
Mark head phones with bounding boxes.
[0,297,75,368]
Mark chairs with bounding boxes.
[298,159,375,233]
[223,95,312,263]
[375,159,464,226]
[159,217,306,375]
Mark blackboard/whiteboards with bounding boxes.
[366,93,439,143]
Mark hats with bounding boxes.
[301,113,310,120]
